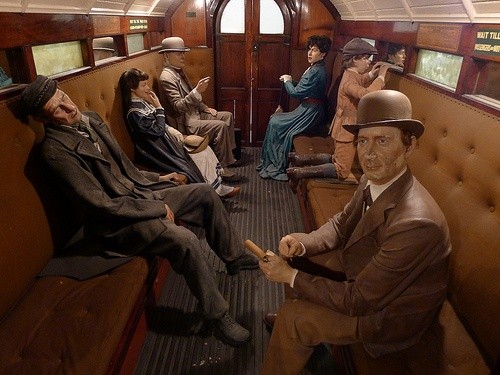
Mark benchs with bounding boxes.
[288,47,500,375]
[0,45,215,374]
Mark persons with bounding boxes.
[92,37,115,61]
[158,36,244,183]
[119,67,241,197]
[19,74,266,345]
[255,35,333,181]
[286,37,390,194]
[387,42,406,67]
[245,88,454,375]
